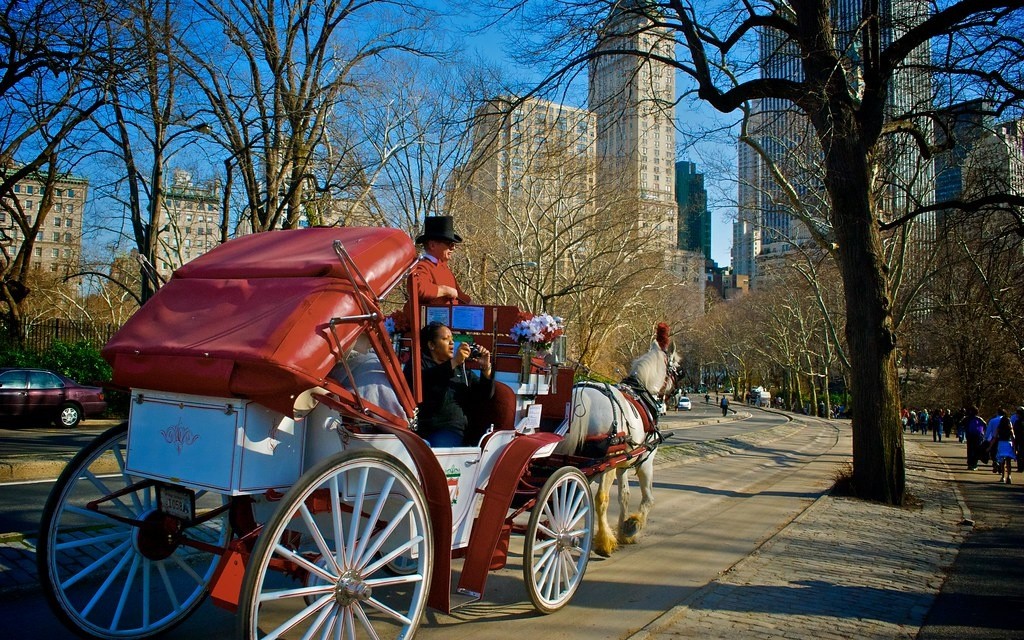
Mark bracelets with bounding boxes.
[483,364,492,372]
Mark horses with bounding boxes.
[555,340,685,558]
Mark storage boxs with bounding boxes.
[123,387,307,496]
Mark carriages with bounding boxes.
[35,229,686,640]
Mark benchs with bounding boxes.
[398,301,555,396]
[314,380,518,501]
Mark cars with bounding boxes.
[677,397,693,411]
[751,386,758,392]
[724,387,733,393]
[682,386,693,394]
[0,367,108,430]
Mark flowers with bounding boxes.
[510,311,565,357]
[383,309,409,356]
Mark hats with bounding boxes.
[416,216,462,244]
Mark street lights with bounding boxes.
[139,121,213,308]
[495,261,537,305]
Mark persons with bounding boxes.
[772,397,844,418]
[704,393,711,403]
[402,215,471,397]
[720,395,730,416]
[683,391,688,398]
[901,406,988,470]
[986,415,1015,484]
[1011,406,1024,472]
[403,321,495,448]
[746,392,751,404]
[984,407,1008,474]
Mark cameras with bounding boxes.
[469,346,482,359]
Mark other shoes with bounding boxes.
[1006,476,1011,485]
[1000,477,1005,482]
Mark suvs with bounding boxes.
[694,383,709,394]
